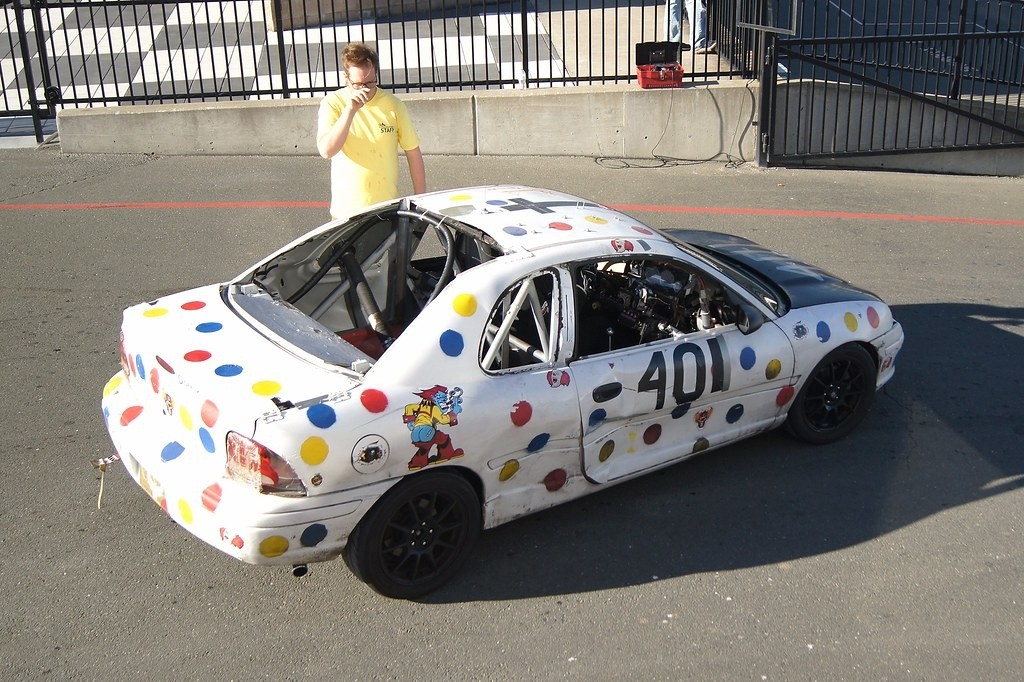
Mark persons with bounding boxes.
[664,0,716,54]
[316,42,426,222]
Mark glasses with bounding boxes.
[348,73,377,90]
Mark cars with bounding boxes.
[99,182,907,600]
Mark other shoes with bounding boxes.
[681,42,691,50]
[694,40,718,53]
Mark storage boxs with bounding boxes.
[636,41,684,88]
